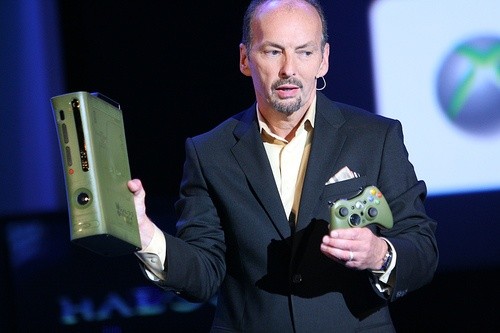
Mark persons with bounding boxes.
[128,0,441,333]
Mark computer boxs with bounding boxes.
[49,92,144,258]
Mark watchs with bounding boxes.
[382,253,392,266]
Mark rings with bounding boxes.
[348,251,353,261]
